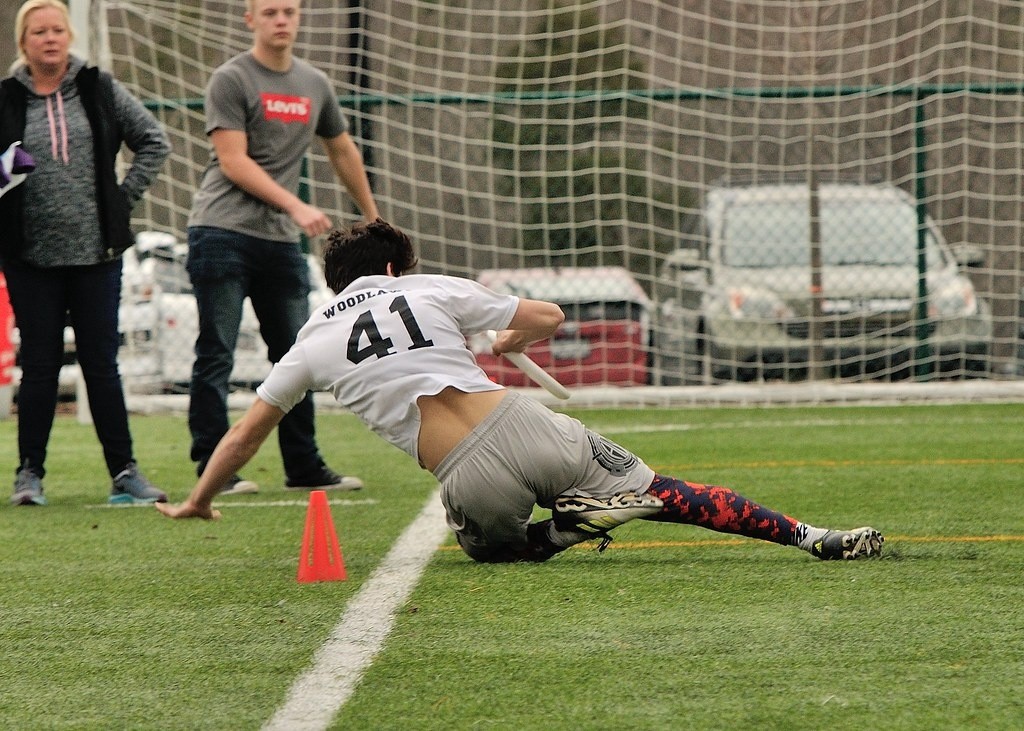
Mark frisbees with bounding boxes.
[486,329,571,400]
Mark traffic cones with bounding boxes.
[296,490,346,583]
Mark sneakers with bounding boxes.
[218,475,259,494]
[812,526,885,560]
[286,465,364,490]
[552,487,665,552]
[109,462,168,503]
[10,470,45,506]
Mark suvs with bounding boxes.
[648,177,991,386]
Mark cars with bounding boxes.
[470,264,653,388]
[0,232,335,415]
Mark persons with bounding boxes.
[186,0,381,493]
[154,213,883,564]
[0,0,172,506]
